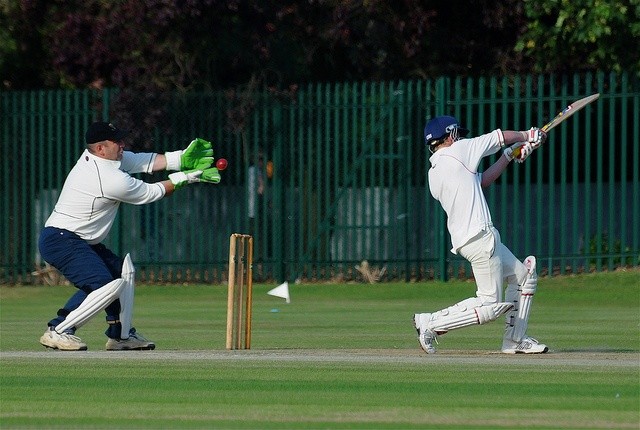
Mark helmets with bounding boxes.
[424,116,470,141]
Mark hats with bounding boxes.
[85,121,129,144]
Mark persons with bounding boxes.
[413,116,550,354]
[38,121,220,351]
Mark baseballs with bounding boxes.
[216,158,227,170]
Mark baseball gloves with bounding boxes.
[167,167,221,193]
[165,138,221,170]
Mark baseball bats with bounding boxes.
[511,93,600,157]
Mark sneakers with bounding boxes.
[501,332,548,355]
[38,326,87,350]
[413,312,440,356]
[105,327,155,351]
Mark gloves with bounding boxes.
[168,167,221,189]
[524,127,548,148]
[504,140,532,164]
[165,138,215,167]
